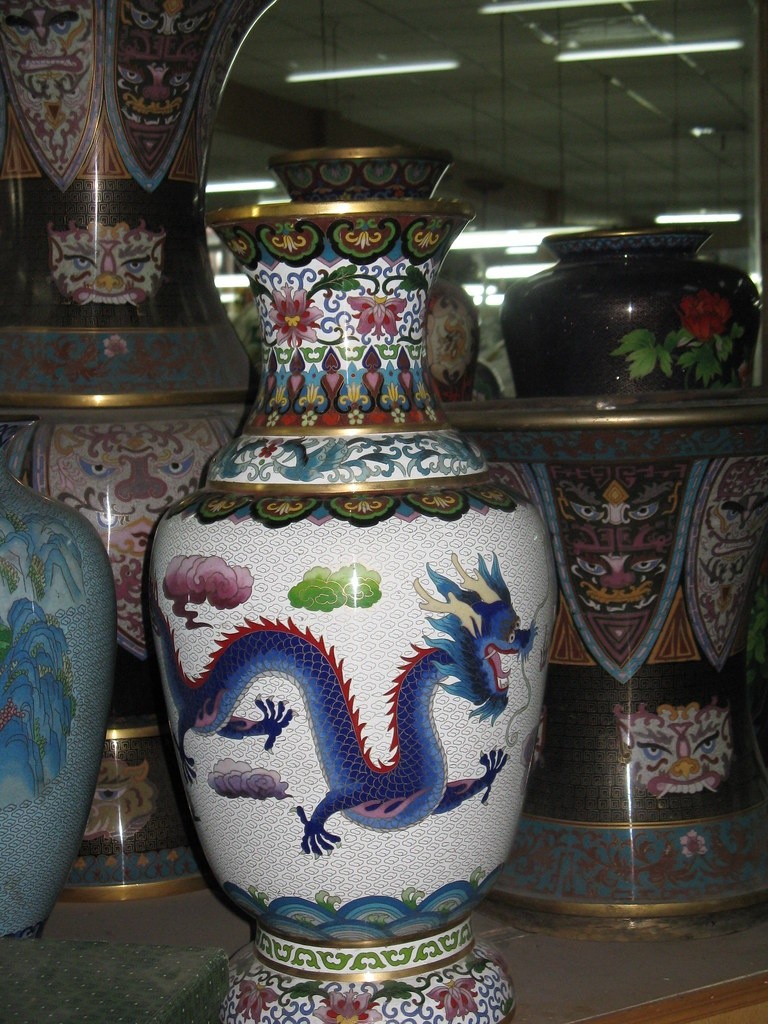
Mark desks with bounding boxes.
[42,885,768,1024]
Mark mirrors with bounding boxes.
[195,1,766,427]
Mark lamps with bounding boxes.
[204,1,744,306]
